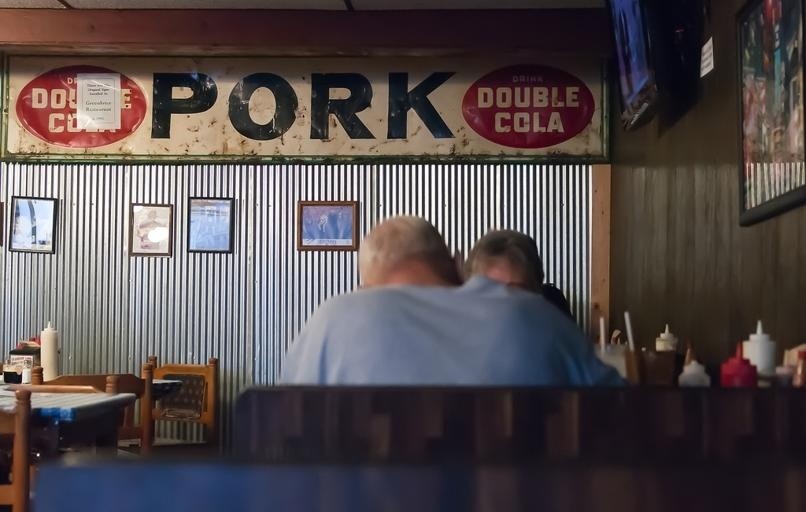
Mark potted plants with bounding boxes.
[297,200,360,251]
[8,195,58,254]
[187,197,235,253]
[733,1,806,229]
[128,202,173,258]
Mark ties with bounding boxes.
[607,0,704,130]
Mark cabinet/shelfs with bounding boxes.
[656,321,793,388]
[40,321,59,382]
[22,360,32,384]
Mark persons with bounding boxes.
[459,225,571,322]
[277,212,632,391]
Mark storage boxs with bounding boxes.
[28,454,806,512]
[231,384,806,512]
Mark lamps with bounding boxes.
[118,356,219,454]
[32,361,154,456]
[4,375,118,462]
[0,389,32,512]
